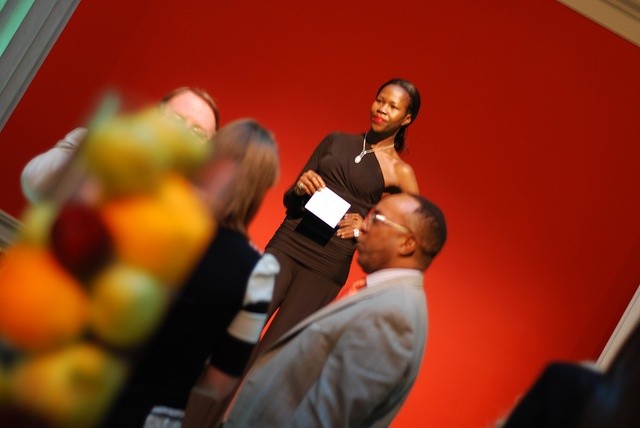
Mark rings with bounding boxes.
[353,229,360,238]
[298,181,303,190]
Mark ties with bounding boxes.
[342,278,367,299]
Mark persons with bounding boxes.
[261,77,420,335]
[114,119,280,427]
[21,85,219,207]
[223,193,448,427]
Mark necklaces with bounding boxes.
[354,128,396,163]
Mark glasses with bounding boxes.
[370,210,414,237]
